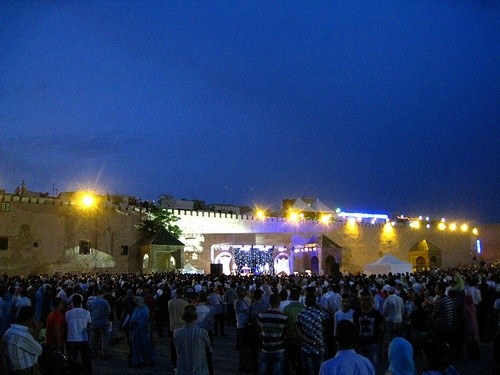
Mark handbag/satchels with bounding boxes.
[244,318,255,331]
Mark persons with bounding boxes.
[45,297,65,375]
[256,294,288,375]
[295,292,331,375]
[332,293,356,340]
[0,262,500,375]
[167,285,190,366]
[65,295,93,375]
[352,290,390,367]
[384,336,417,375]
[0,305,43,375]
[234,286,255,375]
[89,289,112,358]
[383,286,405,337]
[128,296,158,367]
[193,293,214,375]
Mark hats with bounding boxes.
[236,287,247,293]
[64,279,74,288]
[79,284,88,290]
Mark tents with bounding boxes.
[179,262,204,274]
[363,255,413,275]
